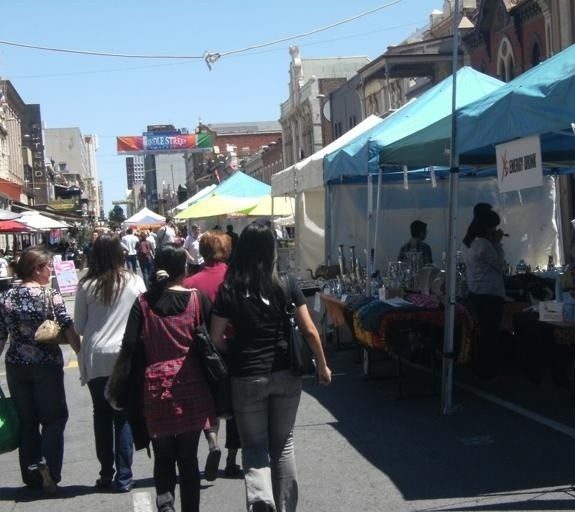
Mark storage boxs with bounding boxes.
[539,299,575,327]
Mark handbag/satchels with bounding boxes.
[34,287,70,344]
[277,267,321,379]
[196,287,231,384]
[0,385,21,455]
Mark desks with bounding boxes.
[320,282,476,402]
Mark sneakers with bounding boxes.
[225,462,243,481]
[205,446,221,482]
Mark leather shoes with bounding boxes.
[96,480,113,491]
[38,462,57,495]
[118,481,136,492]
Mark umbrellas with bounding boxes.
[0,220,29,251]
[15,210,72,232]
[0,207,22,220]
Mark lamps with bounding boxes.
[315,94,335,101]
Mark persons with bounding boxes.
[473,203,494,220]
[182,230,243,481]
[398,220,434,270]
[0,214,238,295]
[464,210,506,382]
[210,221,334,512]
[1,247,83,496]
[103,243,213,512]
[73,234,149,493]
